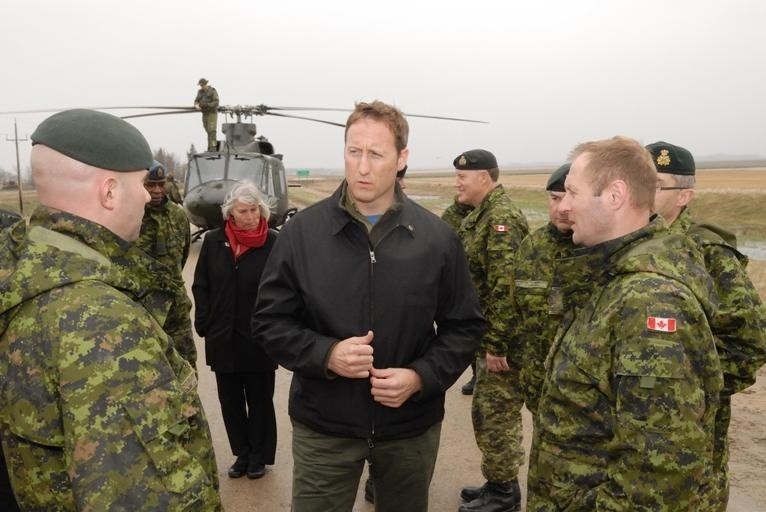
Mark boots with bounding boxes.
[462,363,476,394]
[458,477,521,511]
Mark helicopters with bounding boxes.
[0,104,494,244]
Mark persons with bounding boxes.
[364,164,408,504]
[441,142,585,512]
[251,101,486,510]
[194,78,219,152]
[192,180,280,480]
[135,160,199,382]
[526,136,724,511]
[2,109,225,511]
[645,141,765,511]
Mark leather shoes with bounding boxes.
[228,456,247,477]
[246,459,265,479]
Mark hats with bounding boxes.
[30,108,153,172]
[149,160,167,182]
[646,141,695,176]
[199,79,208,86]
[453,149,497,169]
[546,163,571,192]
